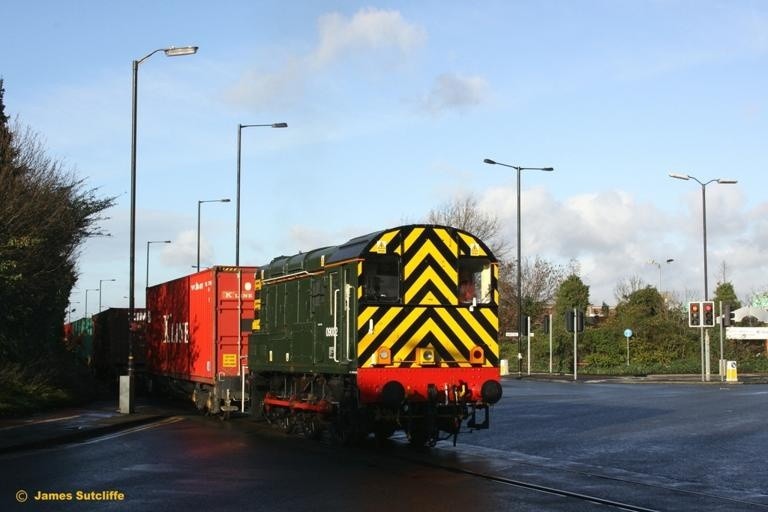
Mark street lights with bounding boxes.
[483,159,555,381]
[236,120,290,269]
[668,172,739,382]
[646,259,675,293]
[145,240,171,288]
[126,45,202,414]
[69,301,80,323]
[84,288,99,317]
[124,296,135,308]
[195,199,234,270]
[98,279,116,312]
[190,265,210,271]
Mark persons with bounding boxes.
[458,271,478,304]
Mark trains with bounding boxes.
[63,224,502,452]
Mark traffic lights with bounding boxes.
[702,299,715,328]
[723,304,735,325]
[688,302,699,327]
